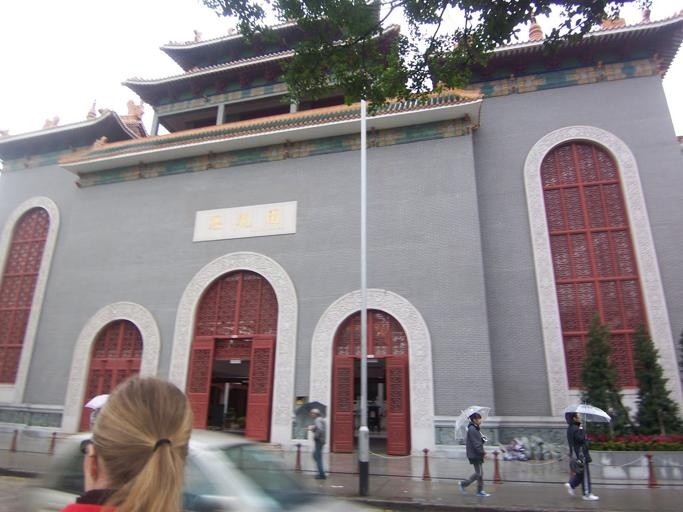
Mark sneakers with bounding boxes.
[458,481,490,497]
[565,483,599,501]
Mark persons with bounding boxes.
[56,373,192,512]
[306,408,330,482]
[562,412,600,501]
[457,412,491,498]
[88,407,99,431]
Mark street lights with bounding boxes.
[333,18,373,503]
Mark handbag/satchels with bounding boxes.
[569,458,584,475]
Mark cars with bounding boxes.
[25,429,392,511]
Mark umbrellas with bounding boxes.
[295,402,327,427]
[452,403,490,443]
[564,400,611,433]
[83,393,110,408]
